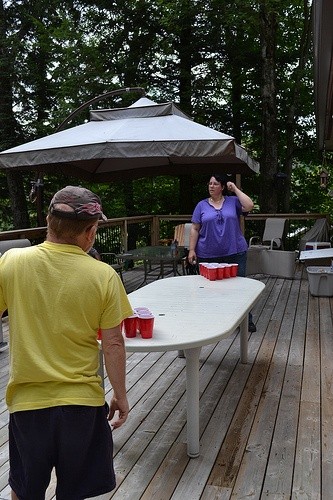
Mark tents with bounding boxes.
[0,87,261,229]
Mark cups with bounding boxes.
[121,322,123,332]
[124,307,155,338]
[97,328,102,340]
[199,262,238,281]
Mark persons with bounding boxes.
[187,175,257,333]
[0,185,128,500]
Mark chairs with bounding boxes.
[249,218,288,250]
[168,224,198,276]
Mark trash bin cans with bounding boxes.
[306,266,333,297]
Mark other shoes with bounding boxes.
[248,321,257,332]
[0,342,9,352]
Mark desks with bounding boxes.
[118,245,185,291]
[98,275,266,457]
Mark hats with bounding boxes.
[47,185,108,223]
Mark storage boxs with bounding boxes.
[307,266,333,296]
